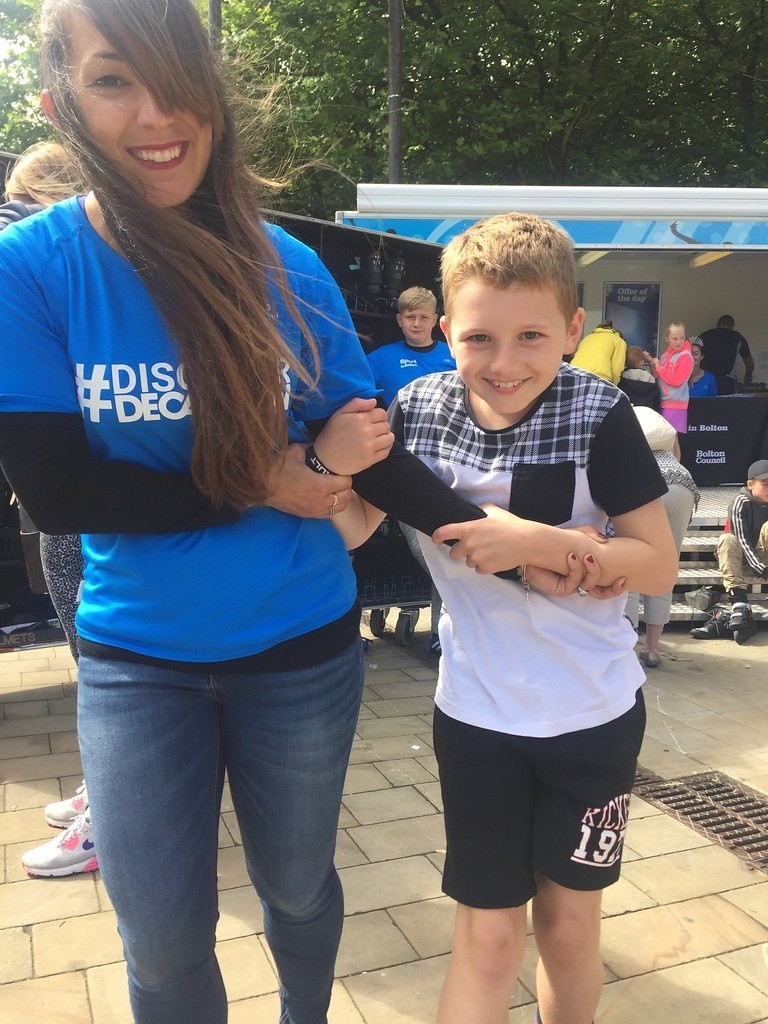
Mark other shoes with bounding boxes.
[639,648,660,667]
[429,637,442,654]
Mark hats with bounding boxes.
[748,459,768,479]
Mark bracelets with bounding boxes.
[521,565,530,590]
[306,447,337,475]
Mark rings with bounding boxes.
[332,494,338,506]
[576,587,588,597]
[329,506,334,516]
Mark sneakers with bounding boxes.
[690,608,730,638]
[44,779,88,828]
[21,806,99,876]
[685,586,721,610]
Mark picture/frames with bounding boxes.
[575,280,662,367]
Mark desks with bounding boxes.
[656,391,768,488]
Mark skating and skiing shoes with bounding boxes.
[728,589,758,644]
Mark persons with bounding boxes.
[569,320,626,386]
[366,286,456,654]
[715,460,768,645]
[643,321,695,460]
[5,145,100,878]
[618,346,660,413]
[698,315,754,394]
[688,336,718,396]
[1,0,626,1023]
[306,213,679,1022]
[607,451,701,668]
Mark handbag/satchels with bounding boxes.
[631,405,676,450]
